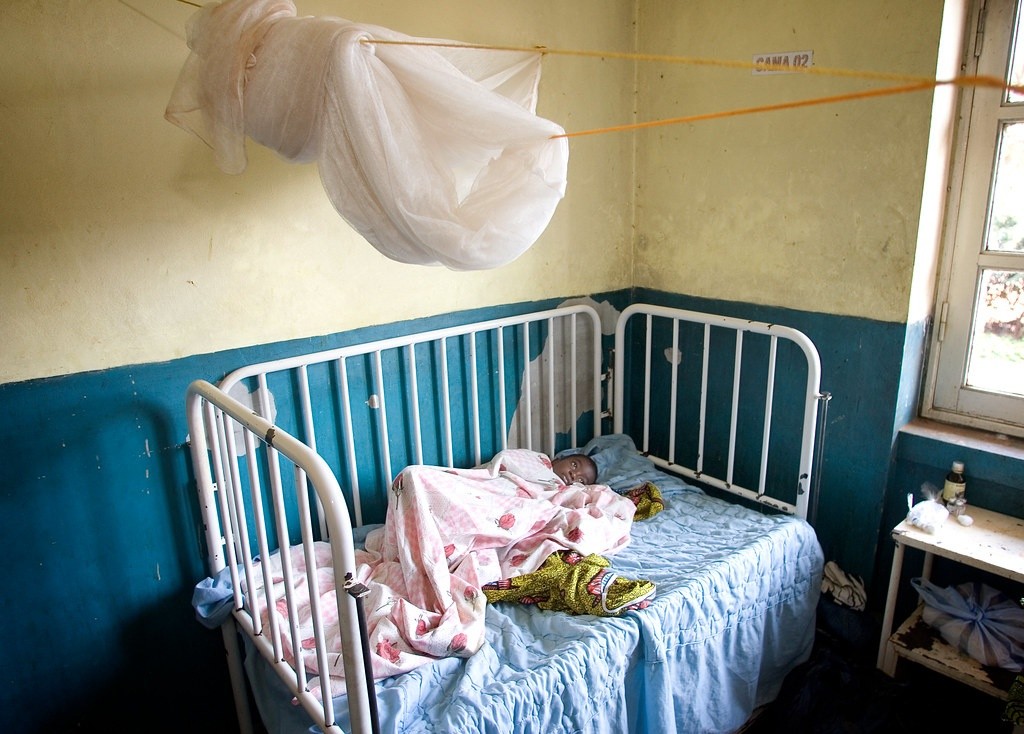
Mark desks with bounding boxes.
[877,496,1024,710]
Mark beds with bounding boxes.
[219,435,802,734]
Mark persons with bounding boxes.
[386,450,598,548]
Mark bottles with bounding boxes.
[942,460,966,508]
